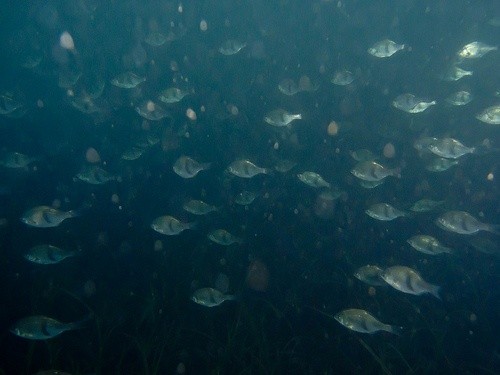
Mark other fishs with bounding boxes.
[0,0,500,348]
